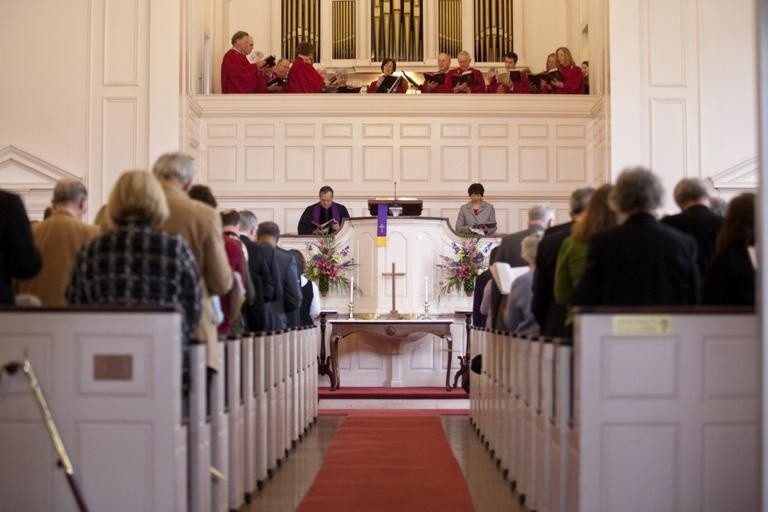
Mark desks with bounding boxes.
[325,316,454,392]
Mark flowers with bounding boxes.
[434,232,493,305]
[302,232,363,299]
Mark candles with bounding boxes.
[424,276,429,304]
[350,276,354,304]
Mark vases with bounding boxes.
[319,273,329,297]
[464,277,474,297]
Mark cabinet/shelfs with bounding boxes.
[451,310,474,394]
[316,309,339,391]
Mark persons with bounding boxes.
[299,186,351,235]
[456,183,497,235]
[1,153,324,421]
[222,31,589,92]
[468,164,756,378]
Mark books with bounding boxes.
[311,219,339,232]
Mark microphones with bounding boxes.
[394,181,396,200]
